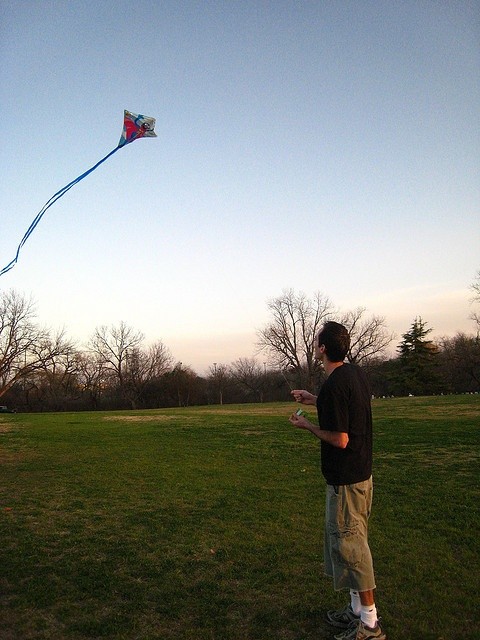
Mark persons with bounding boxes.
[289,321,388,640]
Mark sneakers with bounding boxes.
[333,617,388,640]
[320,601,379,629]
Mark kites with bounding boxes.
[0,110,158,274]
